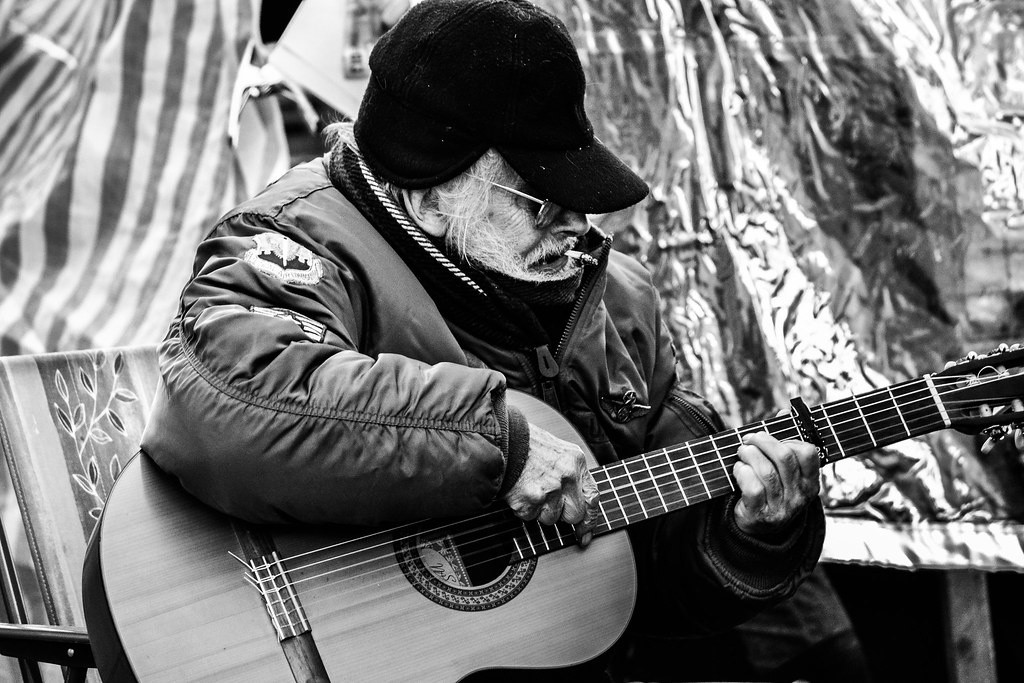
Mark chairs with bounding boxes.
[0,346,164,683]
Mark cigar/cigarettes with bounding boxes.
[564,250,598,265]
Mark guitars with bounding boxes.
[78,341,1024,683]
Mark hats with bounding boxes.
[353,0,650,214]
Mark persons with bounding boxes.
[140,0,865,683]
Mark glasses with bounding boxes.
[461,170,564,229]
